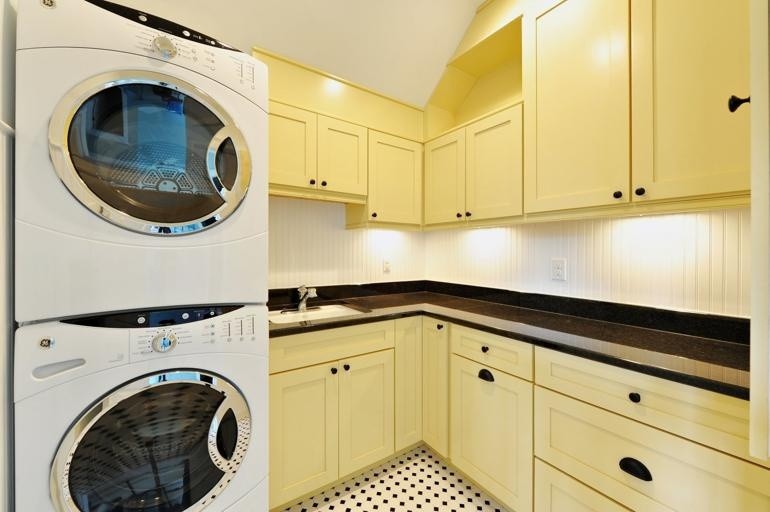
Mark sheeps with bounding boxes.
[297,284,318,312]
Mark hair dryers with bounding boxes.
[12,303,271,512]
[14,0,270,323]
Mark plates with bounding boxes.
[532,338,769,510]
[248,41,370,209]
[271,314,421,512]
[520,1,751,225]
[421,1,527,234]
[418,309,534,512]
[345,87,428,231]
